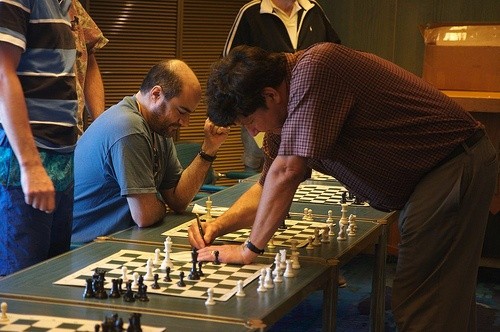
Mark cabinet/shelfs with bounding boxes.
[388,92,500,267]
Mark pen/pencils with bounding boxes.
[194,210,205,244]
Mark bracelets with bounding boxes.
[198,150,218,163]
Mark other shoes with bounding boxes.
[358,286,394,314]
[338,270,348,290]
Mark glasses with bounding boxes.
[152,148,161,180]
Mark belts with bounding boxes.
[426,123,486,173]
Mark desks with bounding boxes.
[0,167,400,332]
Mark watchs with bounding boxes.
[242,237,265,257]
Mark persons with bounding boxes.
[0,0,76,276]
[68,58,231,251]
[69,0,105,141]
[189,44,500,331]
[222,0,342,177]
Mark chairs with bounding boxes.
[174,142,260,193]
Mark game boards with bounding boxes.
[310,168,338,181]
[0,312,166,332]
[53,249,275,302]
[161,214,337,247]
[292,185,370,206]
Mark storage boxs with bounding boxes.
[419,22,500,92]
